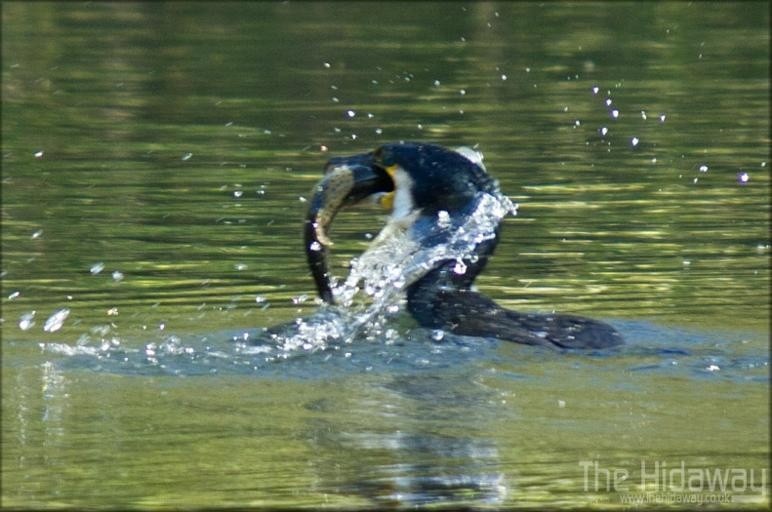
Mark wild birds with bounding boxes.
[323,143,627,350]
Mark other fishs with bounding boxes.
[303,144,488,305]
